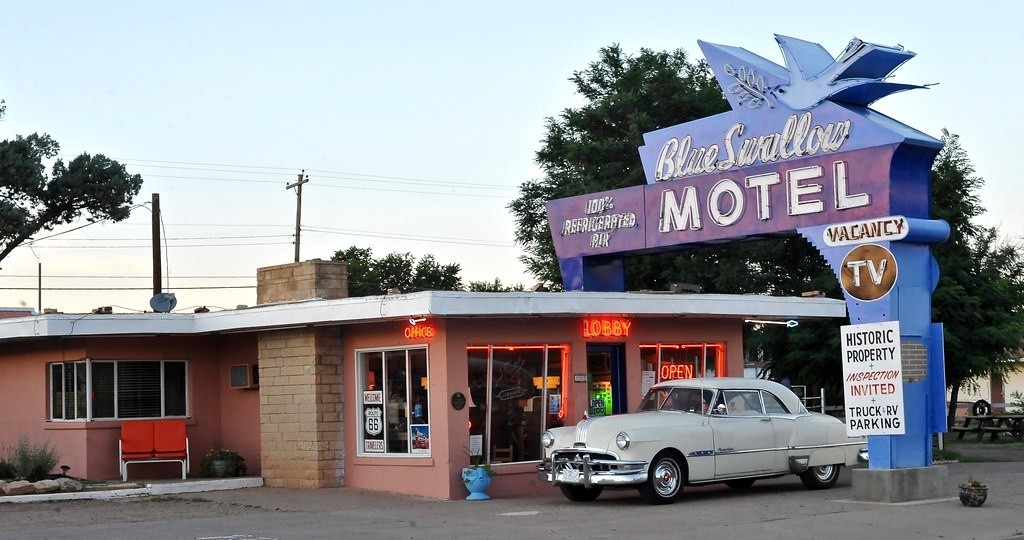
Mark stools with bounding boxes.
[950,426,1013,434]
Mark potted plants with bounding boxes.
[461,445,495,501]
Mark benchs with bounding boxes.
[119,419,190,481]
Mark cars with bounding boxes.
[538,377,869,505]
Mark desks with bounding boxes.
[950,414,1024,444]
